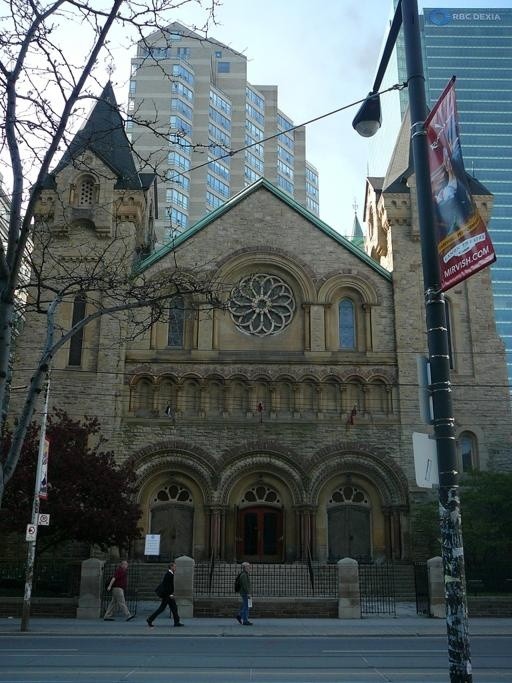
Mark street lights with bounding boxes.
[21,362,54,633]
[352,0,473,683]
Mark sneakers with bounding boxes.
[126,615,136,622]
[243,621,254,626]
[235,613,242,625]
[146,618,155,627]
[173,620,185,627]
[103,617,116,622]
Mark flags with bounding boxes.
[423,73,498,293]
[38,435,52,501]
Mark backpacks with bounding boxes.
[234,572,249,593]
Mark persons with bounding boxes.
[441,146,459,201]
[103,560,135,622]
[236,561,253,625]
[146,561,185,627]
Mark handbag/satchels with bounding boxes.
[155,573,166,599]
